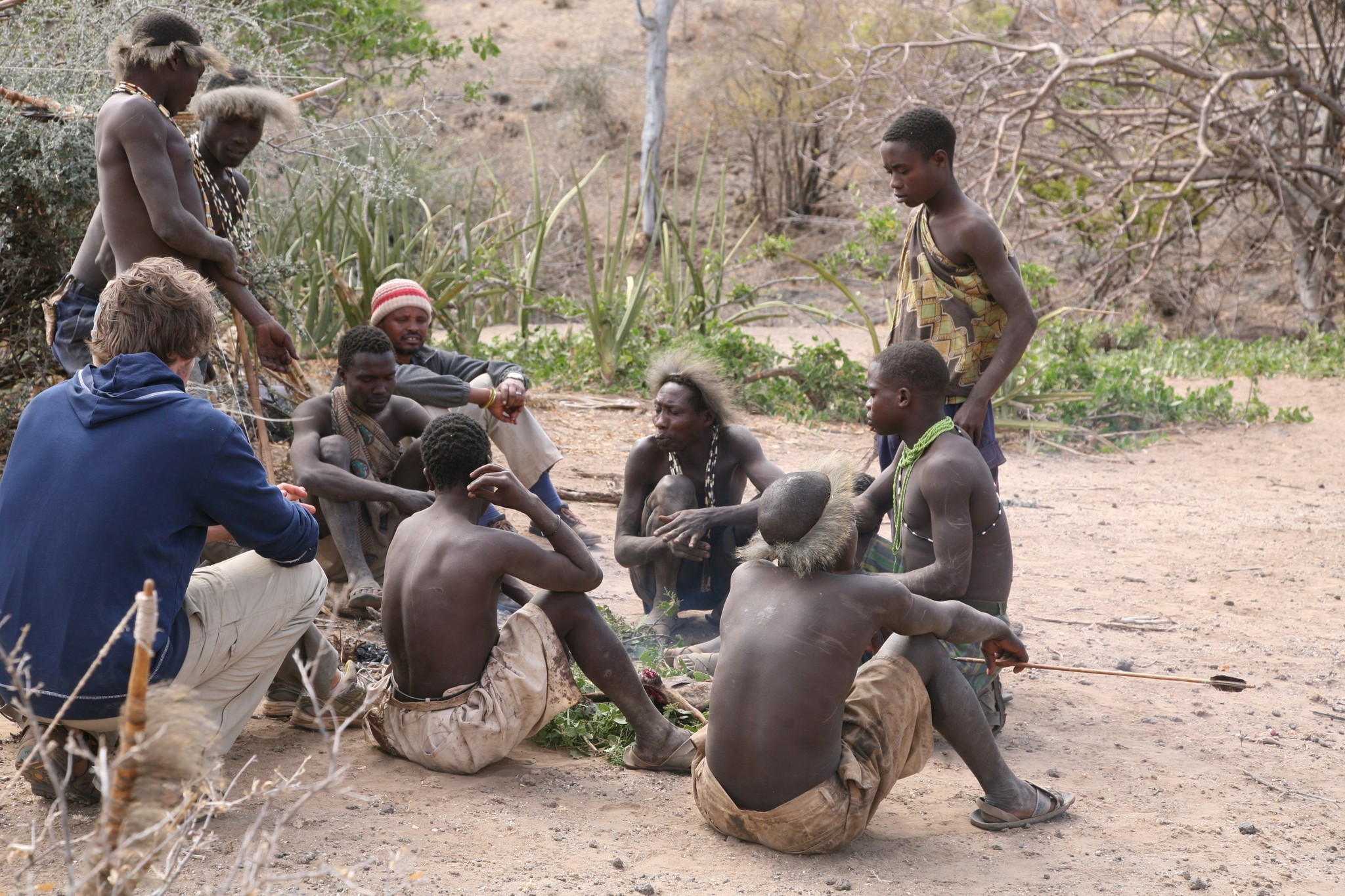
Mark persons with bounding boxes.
[95,12,302,397]
[372,411,698,781]
[290,322,536,609]
[689,470,1079,856]
[609,352,783,650]
[841,341,1017,735]
[2,257,328,820]
[330,277,604,551]
[197,503,372,730]
[50,67,269,410]
[871,109,1040,518]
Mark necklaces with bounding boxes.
[189,132,254,256]
[890,417,954,554]
[111,81,212,234]
[667,425,720,592]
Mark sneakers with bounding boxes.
[289,659,372,731]
[263,676,304,717]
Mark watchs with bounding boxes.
[504,372,526,383]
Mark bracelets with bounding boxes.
[539,515,562,537]
[479,386,497,409]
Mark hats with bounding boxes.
[370,278,432,327]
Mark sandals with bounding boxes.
[705,602,723,628]
[973,674,1006,736]
[347,581,382,610]
[661,645,703,668]
[623,729,697,774]
[15,721,101,805]
[673,653,715,679]
[969,779,1076,830]
[634,617,689,648]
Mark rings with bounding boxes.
[516,392,522,396]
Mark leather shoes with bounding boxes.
[528,509,601,546]
